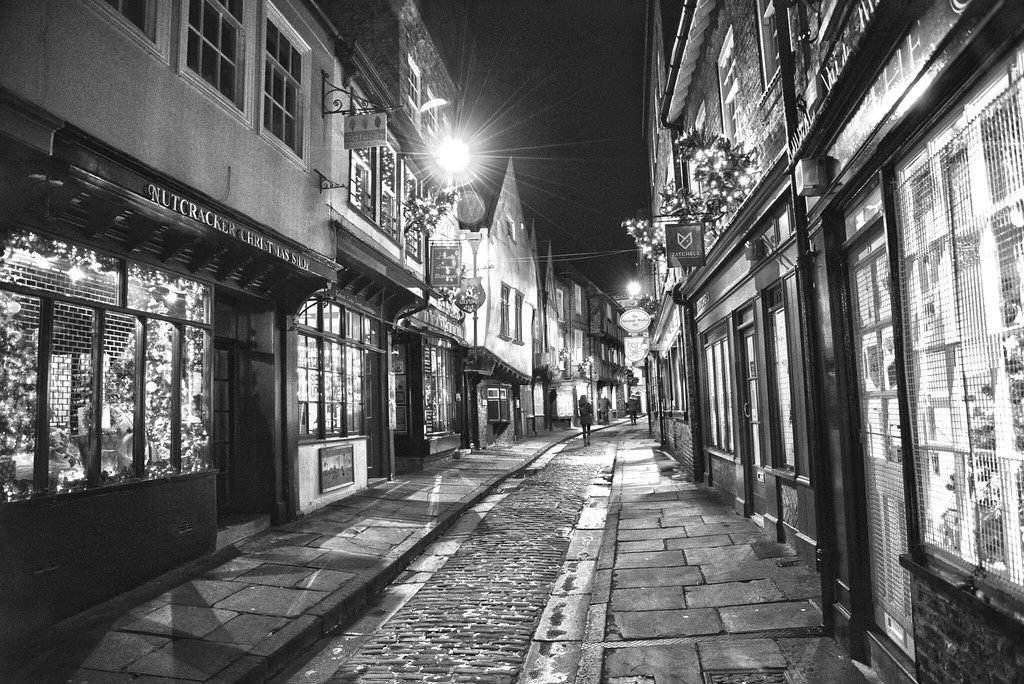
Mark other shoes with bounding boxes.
[606,424,608,426]
[634,424,637,425]
[584,442,587,447]
[587,441,590,445]
[603,424,605,426]
[631,424,633,426]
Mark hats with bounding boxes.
[631,393,636,397]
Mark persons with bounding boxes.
[598,394,612,426]
[579,395,593,447]
[627,395,639,426]
